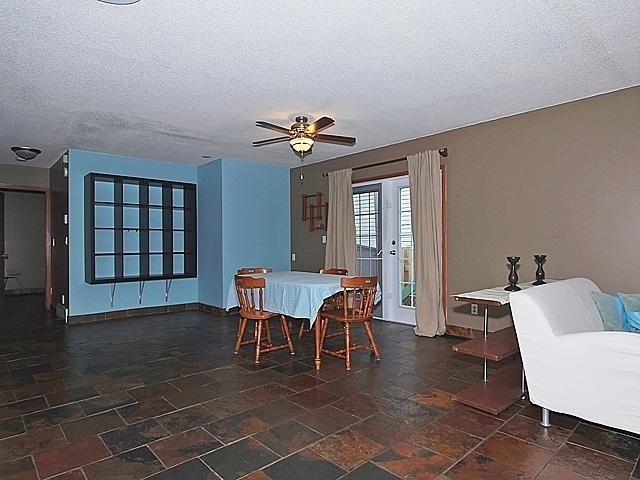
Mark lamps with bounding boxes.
[289,137,315,153]
[11,147,42,160]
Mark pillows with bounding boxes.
[590,290,640,332]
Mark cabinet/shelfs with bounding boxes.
[449,278,559,416]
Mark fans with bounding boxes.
[253,116,355,155]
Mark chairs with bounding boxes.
[234,268,381,371]
[508,276,640,442]
